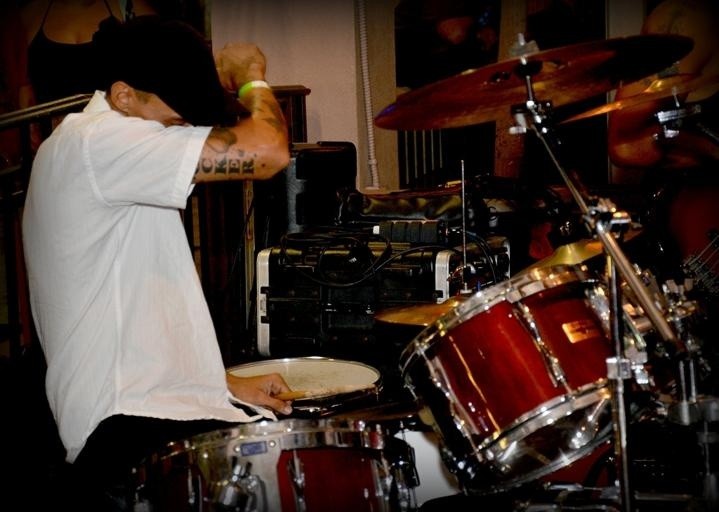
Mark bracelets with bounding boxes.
[234,77,275,100]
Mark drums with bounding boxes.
[133,417,420,511]
[224,353,384,417]
[394,263,638,498]
[548,439,616,487]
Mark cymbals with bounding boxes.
[512,229,643,277]
[559,70,719,125]
[372,32,694,132]
[374,296,471,329]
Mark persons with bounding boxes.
[9,1,164,163]
[15,9,294,511]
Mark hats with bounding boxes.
[103,15,251,126]
